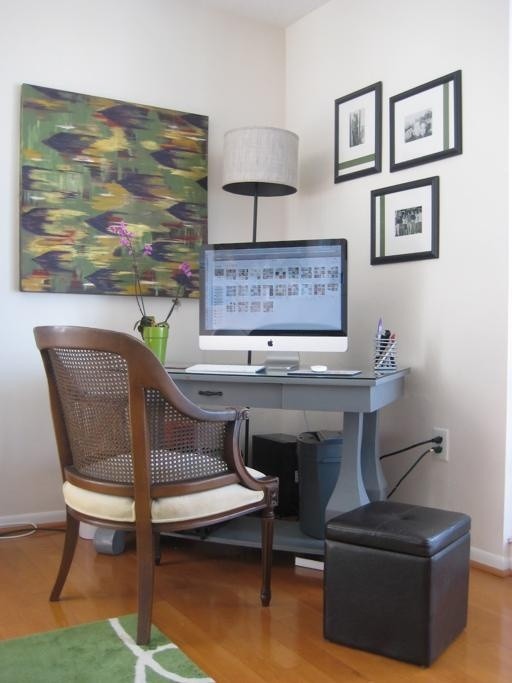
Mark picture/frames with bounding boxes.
[331,65,463,264]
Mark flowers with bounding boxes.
[106,218,197,339]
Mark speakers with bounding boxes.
[250,432,297,519]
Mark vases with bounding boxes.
[143,326,168,365]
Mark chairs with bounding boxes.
[33,324,280,605]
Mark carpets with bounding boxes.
[1,612,216,683]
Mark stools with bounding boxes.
[321,499,470,666]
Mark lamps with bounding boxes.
[221,127,299,240]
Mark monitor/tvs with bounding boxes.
[198,238,349,373]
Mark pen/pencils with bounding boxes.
[375,317,397,368]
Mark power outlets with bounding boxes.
[430,426,449,462]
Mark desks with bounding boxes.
[94,366,411,576]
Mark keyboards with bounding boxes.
[185,361,266,375]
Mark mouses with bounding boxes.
[311,364,329,372]
